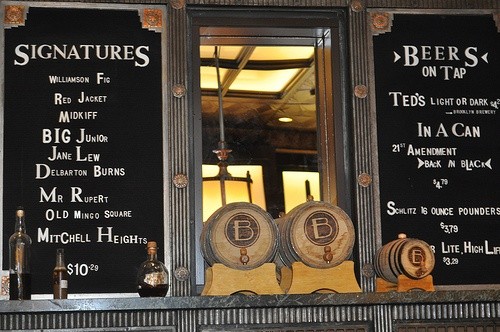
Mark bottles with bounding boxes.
[53,248,68,300]
[8,206,31,300]
[136,241,169,298]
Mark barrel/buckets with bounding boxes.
[199,201,279,270]
[373,237,434,283]
[270,201,356,286]
